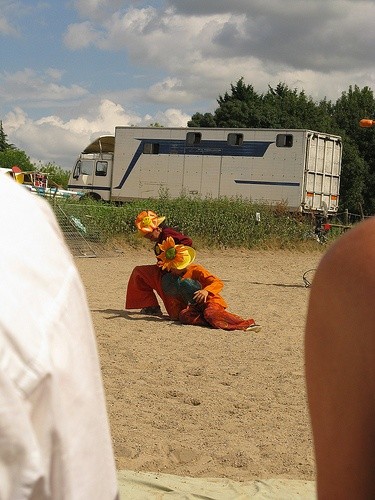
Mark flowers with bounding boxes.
[156,236,190,272]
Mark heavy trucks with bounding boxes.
[67,125,343,218]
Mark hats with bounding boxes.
[157,236,196,269]
[135,210,166,237]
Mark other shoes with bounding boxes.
[246,324,262,332]
[140,307,163,316]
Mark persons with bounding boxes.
[303,217,375,499]
[125,210,195,317]
[156,236,263,333]
